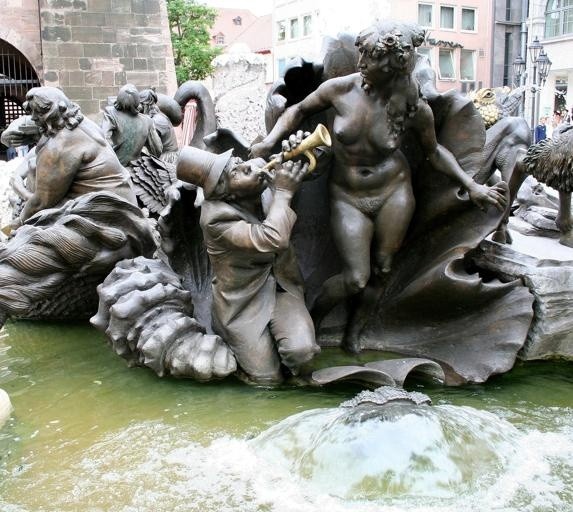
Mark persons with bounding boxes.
[0,16,510,391]
[533,106,572,144]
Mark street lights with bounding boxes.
[512,53,525,90]
[528,35,552,144]
